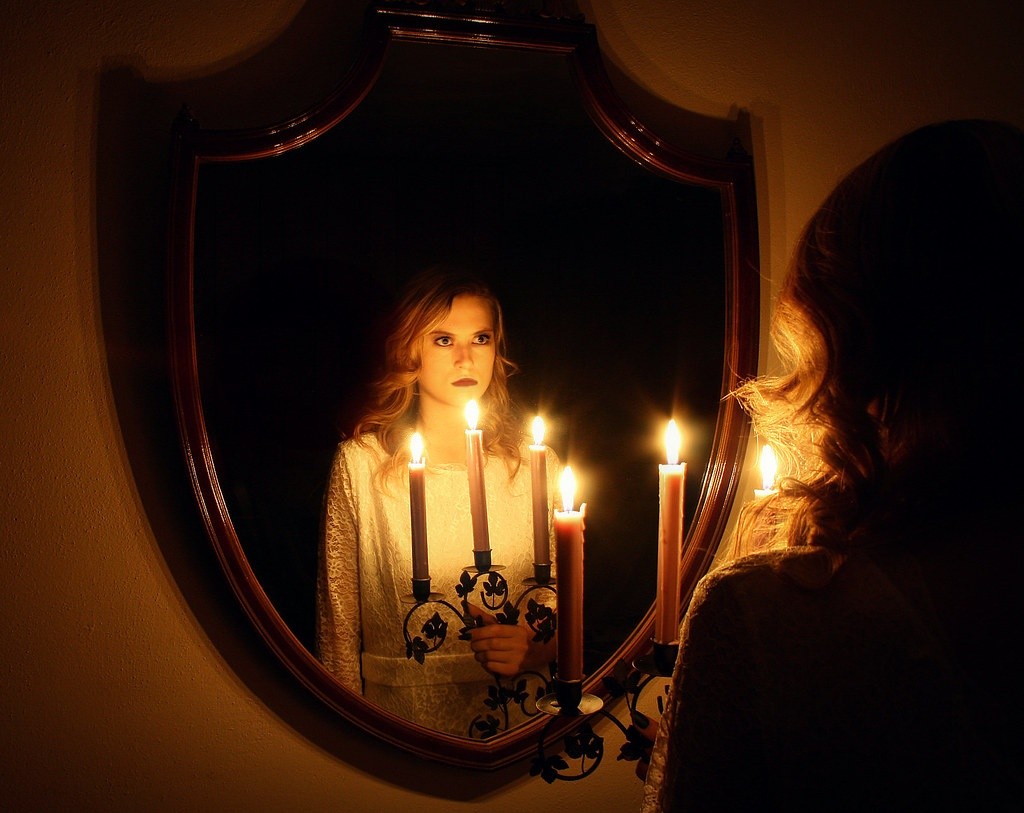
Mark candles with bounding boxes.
[406,432,428,580]
[655,414,689,653]
[461,399,491,551]
[528,414,552,565]
[550,462,588,703]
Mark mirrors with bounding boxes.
[165,1,760,802]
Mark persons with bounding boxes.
[636,120,1024,813]
[319,261,565,742]
